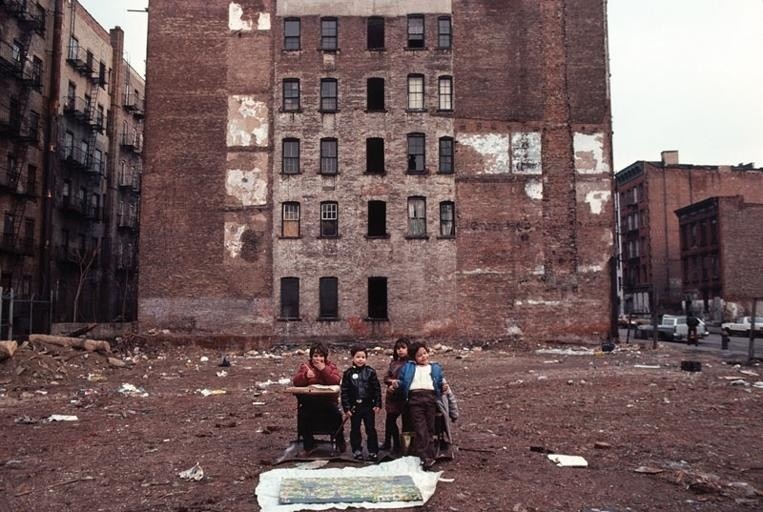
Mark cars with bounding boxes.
[617,313,762,340]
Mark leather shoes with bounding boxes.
[390,449,402,458]
[379,443,391,450]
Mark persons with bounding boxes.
[433,376,459,450]
[292,342,347,457]
[377,336,412,460]
[388,341,450,470]
[686,308,701,347]
[339,342,383,461]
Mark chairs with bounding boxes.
[293,392,336,445]
[435,412,447,441]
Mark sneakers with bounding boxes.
[367,452,378,461]
[335,441,345,452]
[353,450,363,460]
[300,445,317,458]
[423,457,435,471]
